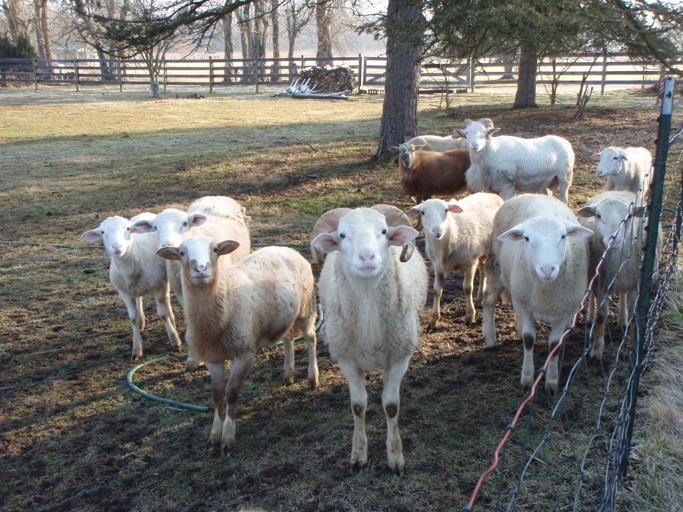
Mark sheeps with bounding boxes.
[589,145,654,192]
[80,211,182,363]
[453,117,577,205]
[406,133,461,151]
[409,191,506,334]
[128,195,252,372]
[479,193,593,392]
[389,142,470,205]
[309,203,429,476]
[156,235,323,449]
[579,191,664,359]
